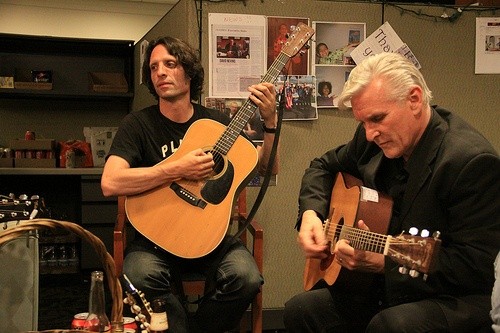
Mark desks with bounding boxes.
[0,167,117,278]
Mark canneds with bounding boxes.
[71,312,100,333]
[109,317,138,333]
[0,131,76,169]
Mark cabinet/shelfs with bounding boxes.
[0,32,136,111]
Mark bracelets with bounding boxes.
[261,122,277,133]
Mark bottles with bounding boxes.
[148,300,171,333]
[83,271,112,333]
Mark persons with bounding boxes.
[246,112,264,139]
[224,39,239,54]
[101,36,281,333]
[221,101,238,118]
[317,81,338,106]
[279,81,313,109]
[317,43,332,64]
[283,52,500,333]
[274,24,292,75]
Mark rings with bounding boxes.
[340,258,343,263]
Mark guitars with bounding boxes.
[302,171,444,292]
[123,22,315,260]
[0,194,49,221]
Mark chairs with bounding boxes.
[113,187,264,333]
